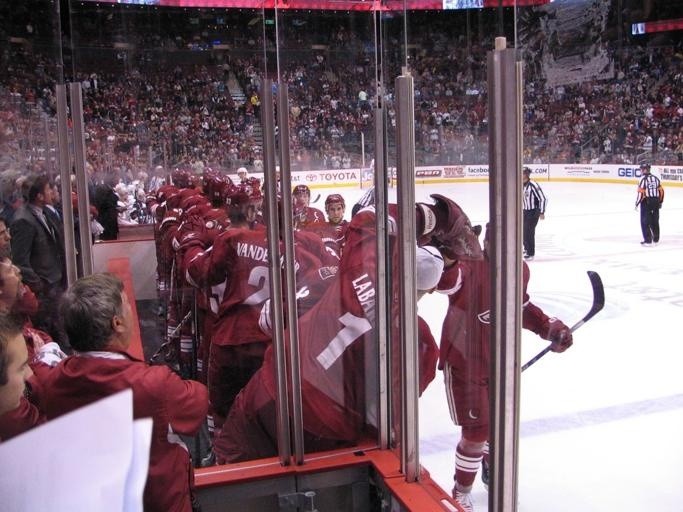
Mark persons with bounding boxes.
[0,1,683,254]
[2,175,572,512]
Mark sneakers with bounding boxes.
[641,238,658,244]
[482,458,490,484]
[452,484,473,512]
[523,250,532,259]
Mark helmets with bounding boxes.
[325,194,345,211]
[294,186,311,194]
[523,168,531,175]
[173,167,261,208]
[640,163,651,169]
[115,180,145,201]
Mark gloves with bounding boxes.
[540,317,573,352]
[145,191,182,233]
[177,216,211,249]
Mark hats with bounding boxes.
[413,245,444,292]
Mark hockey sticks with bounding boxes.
[521,271,604,373]
[310,194,321,204]
